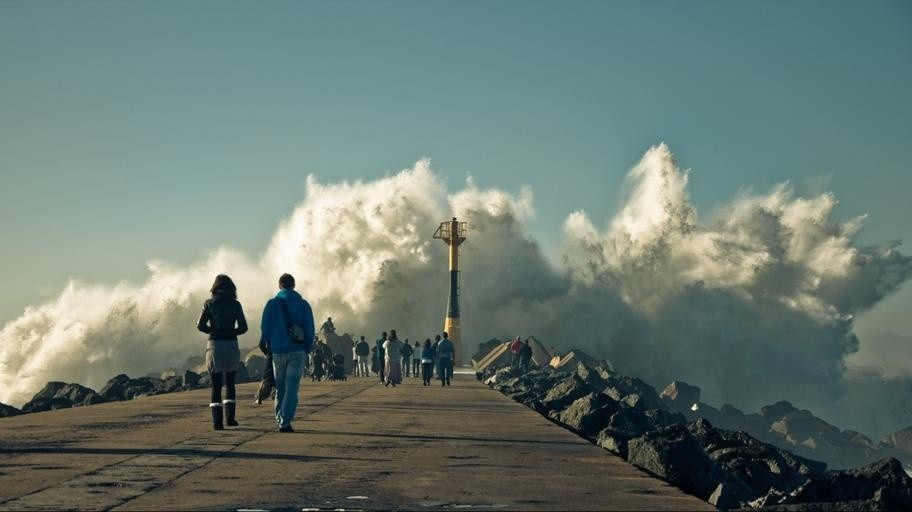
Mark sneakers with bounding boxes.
[381,378,404,389]
[274,415,295,433]
[421,379,451,386]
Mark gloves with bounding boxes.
[258,341,269,357]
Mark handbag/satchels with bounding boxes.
[287,324,307,342]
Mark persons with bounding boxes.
[511,336,524,367]
[305,316,455,388]
[260,273,315,433]
[195,273,248,431]
[518,339,533,370]
[255,336,276,404]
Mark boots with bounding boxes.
[208,402,223,431]
[223,399,238,427]
